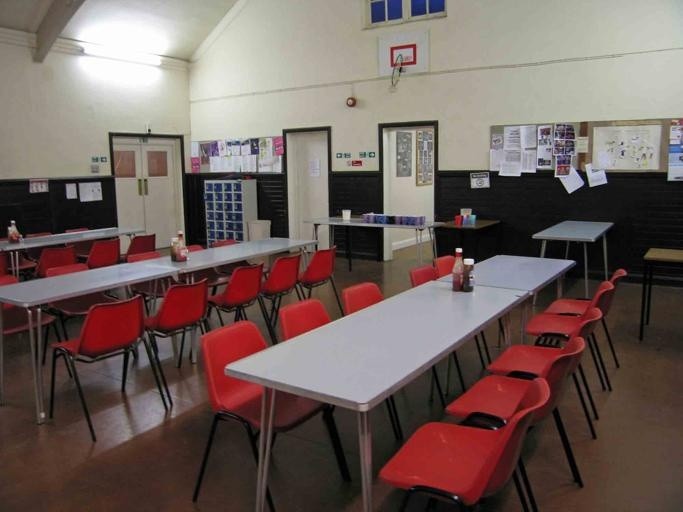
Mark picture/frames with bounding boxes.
[415,128,433,186]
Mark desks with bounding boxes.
[533,218,615,307]
[637,247,683,343]
[303,216,503,271]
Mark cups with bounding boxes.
[455,215,476,227]
[341,210,350,220]
[362,213,425,226]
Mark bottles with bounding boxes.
[461,258,474,292]
[175,231,187,261]
[9,220,19,243]
[451,248,463,290]
[170,238,178,261]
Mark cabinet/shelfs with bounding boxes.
[202,179,260,247]
